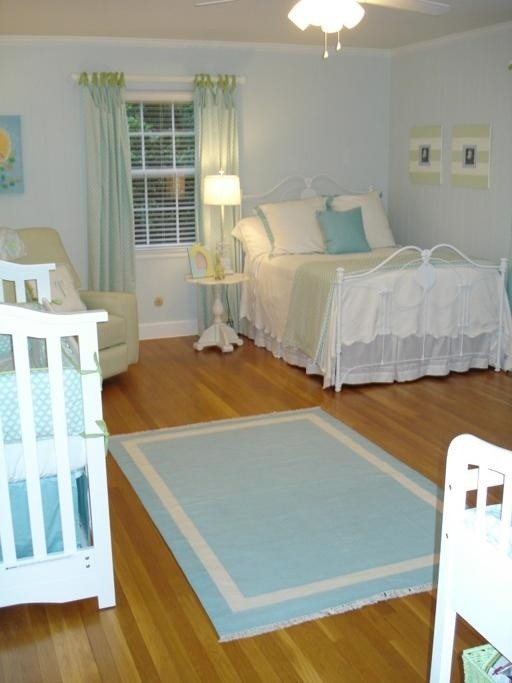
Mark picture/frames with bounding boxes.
[450,121,493,188]
[187,245,214,278]
[0,114,25,195]
[407,125,443,186]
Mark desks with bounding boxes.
[185,272,250,353]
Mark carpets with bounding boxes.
[107,404,445,643]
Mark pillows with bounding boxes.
[25,259,86,313]
[232,190,395,261]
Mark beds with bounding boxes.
[426,433,512,683]
[234,173,512,394]
[0,259,117,609]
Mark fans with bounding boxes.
[195,0,452,18]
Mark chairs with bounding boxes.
[0,224,139,380]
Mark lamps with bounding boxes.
[288,0,365,59]
[203,167,242,275]
